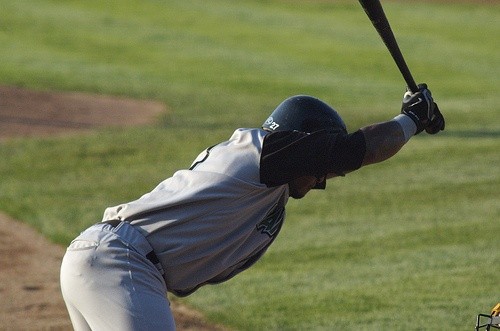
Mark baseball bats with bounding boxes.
[360,0,445,135]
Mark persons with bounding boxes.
[59,80,446,331]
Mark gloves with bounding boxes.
[401,83,437,135]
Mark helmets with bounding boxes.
[261,95,348,133]
